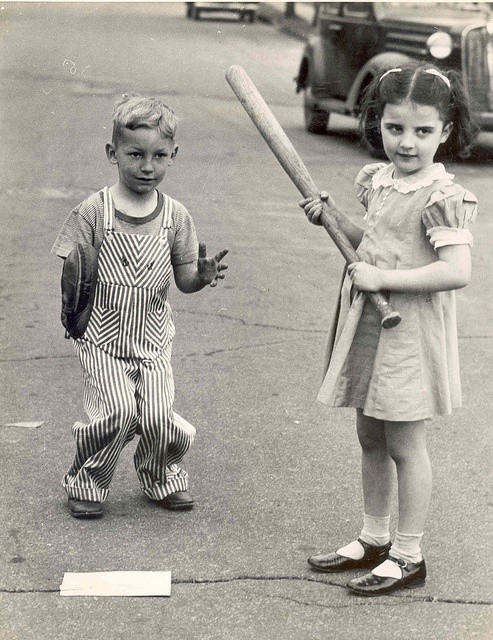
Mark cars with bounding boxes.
[186,2,262,26]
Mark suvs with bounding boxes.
[294,2,493,136]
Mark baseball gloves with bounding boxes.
[62,242,98,341]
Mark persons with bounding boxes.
[300,60,479,597]
[51,95,228,518]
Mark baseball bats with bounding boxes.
[225,65,400,329]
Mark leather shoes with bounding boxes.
[158,490,193,511]
[67,498,103,518]
[346,556,425,596]
[307,538,392,571]
